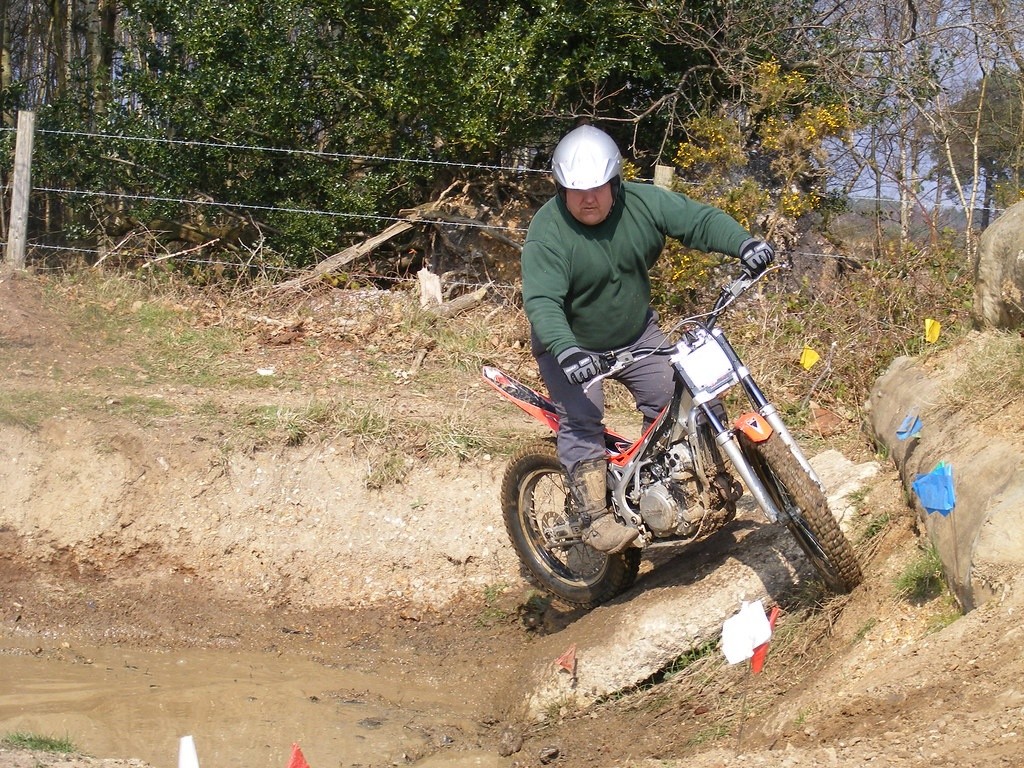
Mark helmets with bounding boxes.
[552,124,623,201]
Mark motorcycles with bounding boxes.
[479,259,866,614]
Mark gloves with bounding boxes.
[557,346,599,385]
[739,238,774,274]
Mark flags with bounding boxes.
[722,600,778,674]
[287,743,310,768]
[800,343,821,371]
[925,319,941,343]
[896,405,923,441]
[912,459,956,517]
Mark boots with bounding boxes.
[565,459,640,555]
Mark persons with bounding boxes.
[522,125,776,556]
